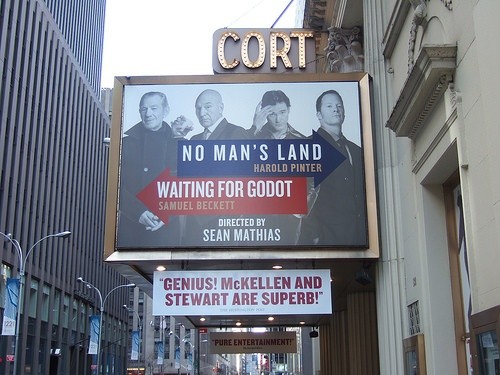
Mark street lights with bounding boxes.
[75,277,136,372]
[0,231,71,372]
[123,304,141,370]
[149,320,184,374]
[168,332,197,375]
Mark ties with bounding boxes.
[337,139,351,164]
[202,129,210,141]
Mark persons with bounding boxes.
[120,90,367,246]
